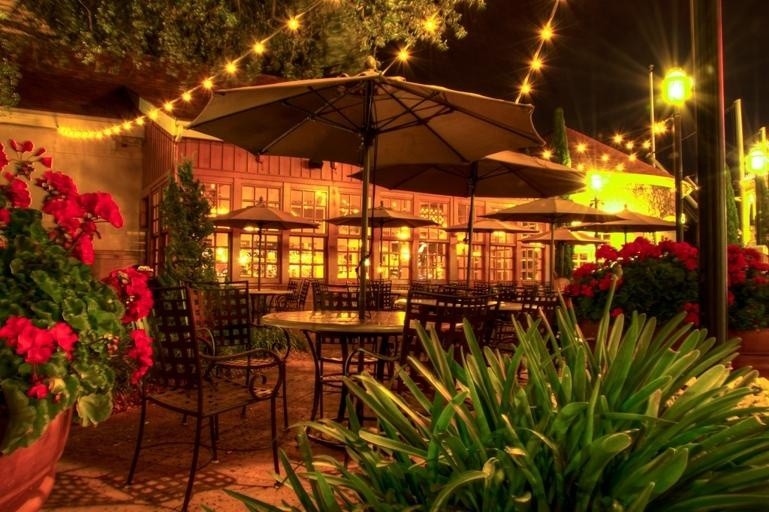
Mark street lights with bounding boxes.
[747,148,768,245]
[662,69,694,242]
[588,173,602,262]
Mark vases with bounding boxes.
[0,403,75,512]
[577,319,768,382]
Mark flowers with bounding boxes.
[0,138,153,458]
[564,237,769,331]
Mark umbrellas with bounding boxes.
[321,201,441,283]
[178,53,551,422]
[343,149,590,292]
[436,215,539,287]
[487,193,630,290]
[205,193,321,328]
[567,203,687,245]
[516,223,610,279]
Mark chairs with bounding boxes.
[180,280,562,450]
[123,284,288,511]
